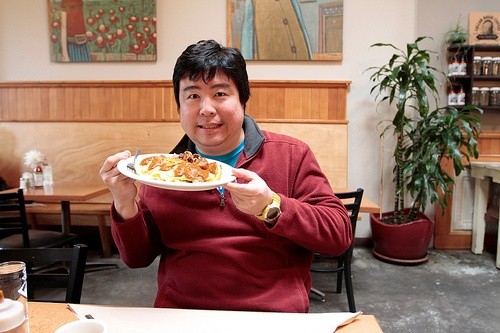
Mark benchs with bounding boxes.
[21,199,112,256]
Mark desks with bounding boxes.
[0,182,112,235]
[469,161,500,270]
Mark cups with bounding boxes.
[42,166,53,185]
[22,173,33,187]
[56,320,104,333]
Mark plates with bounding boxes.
[118,154,238,191]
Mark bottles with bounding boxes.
[448,60,453,76]
[460,59,467,75]
[453,59,460,75]
[457,89,465,105]
[472,88,500,106]
[448,91,457,105]
[25,179,31,190]
[19,178,25,189]
[33,166,43,187]
[473,57,500,75]
[0,290,29,333]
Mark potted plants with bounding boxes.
[437,17,470,61]
[362,36,485,265]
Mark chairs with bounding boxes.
[310,188,366,312]
[0,242,88,304]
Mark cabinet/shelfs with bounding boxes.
[434,43,500,251]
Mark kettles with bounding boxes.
[0,261,28,321]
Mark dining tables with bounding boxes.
[0,301,383,333]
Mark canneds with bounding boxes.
[472,55,500,106]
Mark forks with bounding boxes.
[127,149,140,174]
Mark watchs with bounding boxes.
[256,193,281,223]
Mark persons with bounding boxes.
[98,40,353,315]
